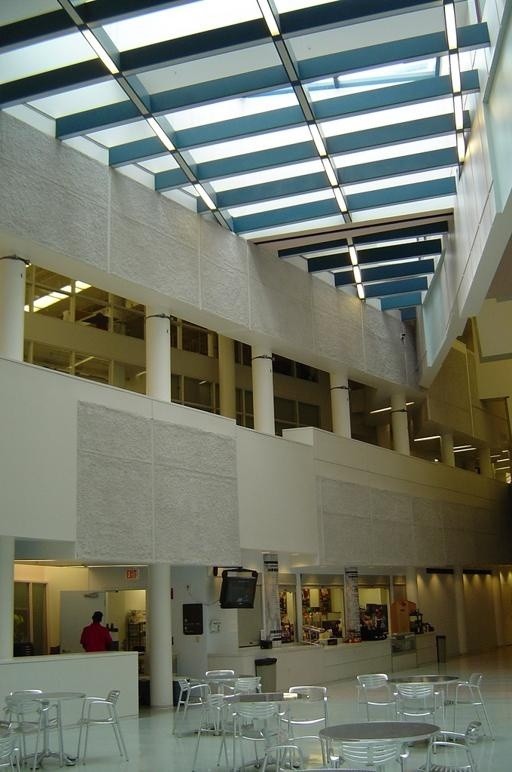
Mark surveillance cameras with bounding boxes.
[401,332,406,339]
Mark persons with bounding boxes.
[80,612,112,651]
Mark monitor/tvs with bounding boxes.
[219,577,257,609]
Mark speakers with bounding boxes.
[183,604,203,635]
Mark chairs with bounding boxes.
[338,694,491,768]
[191,686,332,771]
[171,669,265,735]
[355,670,499,742]
[1,684,128,772]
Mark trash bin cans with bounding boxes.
[255,658,277,693]
[436,635,446,663]
